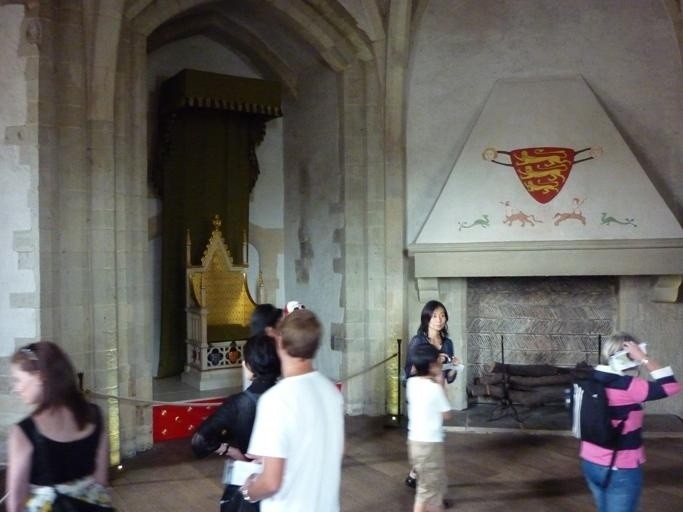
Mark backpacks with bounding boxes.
[569,380,611,441]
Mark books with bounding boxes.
[609,342,647,370]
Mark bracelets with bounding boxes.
[636,354,653,370]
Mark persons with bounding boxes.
[405,342,454,512]
[565,330,681,512]
[400,299,460,509]
[4,340,116,512]
[188,298,346,512]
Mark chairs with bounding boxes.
[177,212,265,391]
